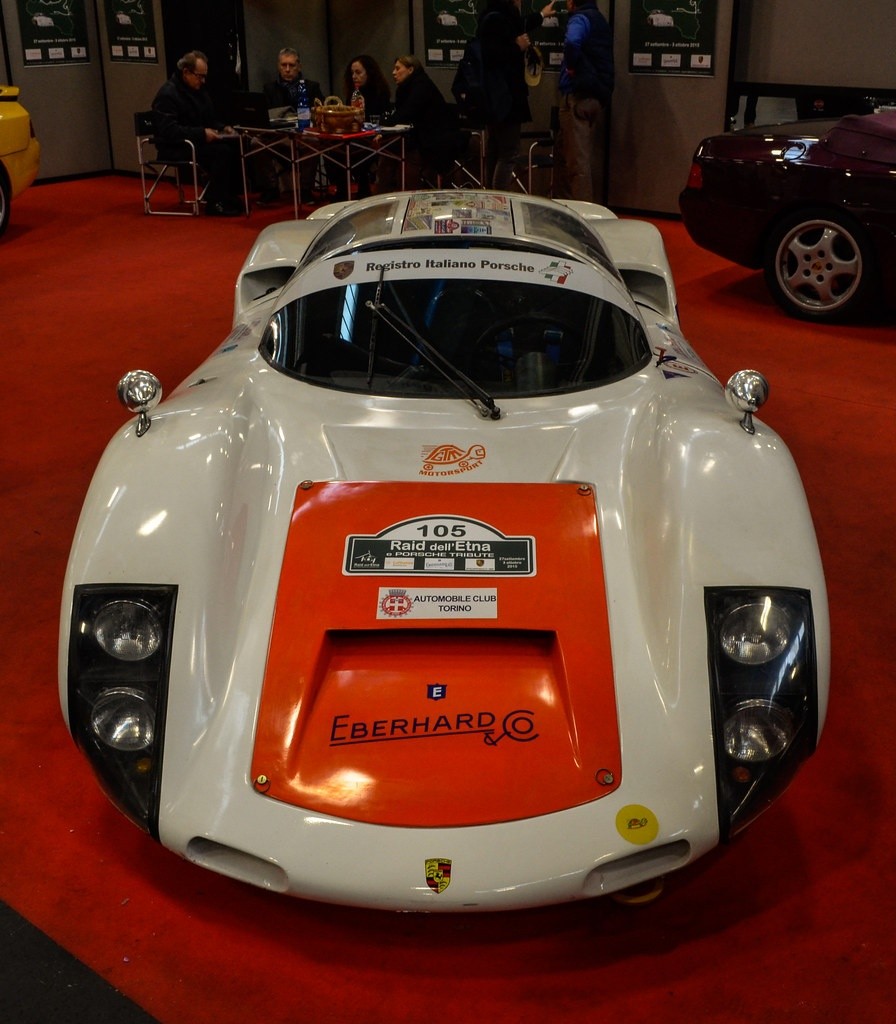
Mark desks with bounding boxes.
[231,124,414,221]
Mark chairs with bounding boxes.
[135,93,559,216]
[410,289,505,384]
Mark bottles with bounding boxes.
[297,80,311,130]
[350,83,365,119]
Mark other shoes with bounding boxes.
[257,190,280,204]
[302,191,315,205]
[205,197,243,215]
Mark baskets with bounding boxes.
[312,96,365,133]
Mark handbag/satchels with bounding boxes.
[450,11,506,123]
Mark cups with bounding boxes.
[370,115,380,125]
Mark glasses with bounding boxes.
[189,71,207,79]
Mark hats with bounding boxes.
[523,45,544,87]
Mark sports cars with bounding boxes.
[51,185,836,915]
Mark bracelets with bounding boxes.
[540,11,544,18]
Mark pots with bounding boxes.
[311,96,363,129]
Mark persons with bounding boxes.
[253,0,613,204]
[149,50,251,215]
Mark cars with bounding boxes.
[0,82,40,238]
[678,105,896,324]
